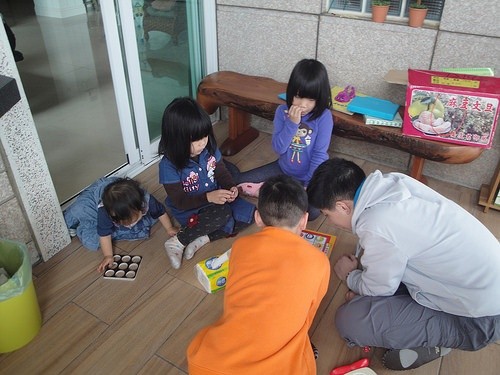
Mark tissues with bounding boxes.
[194,247,232,295]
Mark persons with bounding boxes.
[187,175,331,373]
[307,158,499,371]
[222,59,333,198]
[63,177,182,273]
[159,98,238,269]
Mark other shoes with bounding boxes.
[382,346,452,371]
[236,182,263,197]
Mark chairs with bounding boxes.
[142,0,186,46]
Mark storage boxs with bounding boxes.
[385,68,500,150]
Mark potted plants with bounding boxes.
[408,3,429,27]
[368,0,391,23]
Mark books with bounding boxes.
[278,85,402,127]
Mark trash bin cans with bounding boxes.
[0,237,42,354]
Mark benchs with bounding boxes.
[196,71,484,185]
